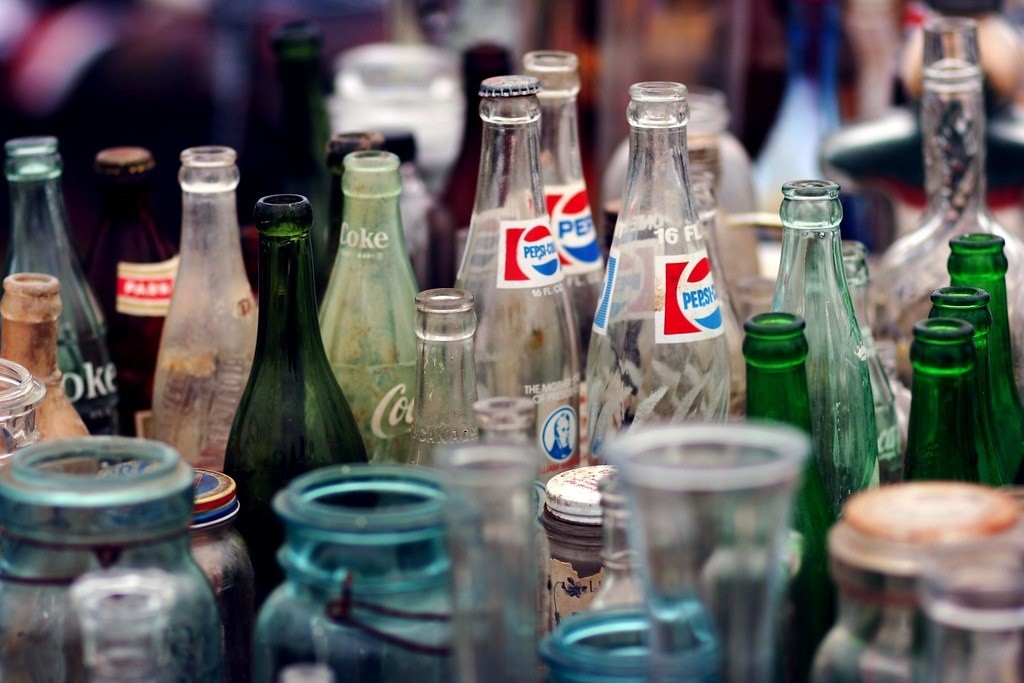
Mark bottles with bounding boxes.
[0,12,1024,681]
